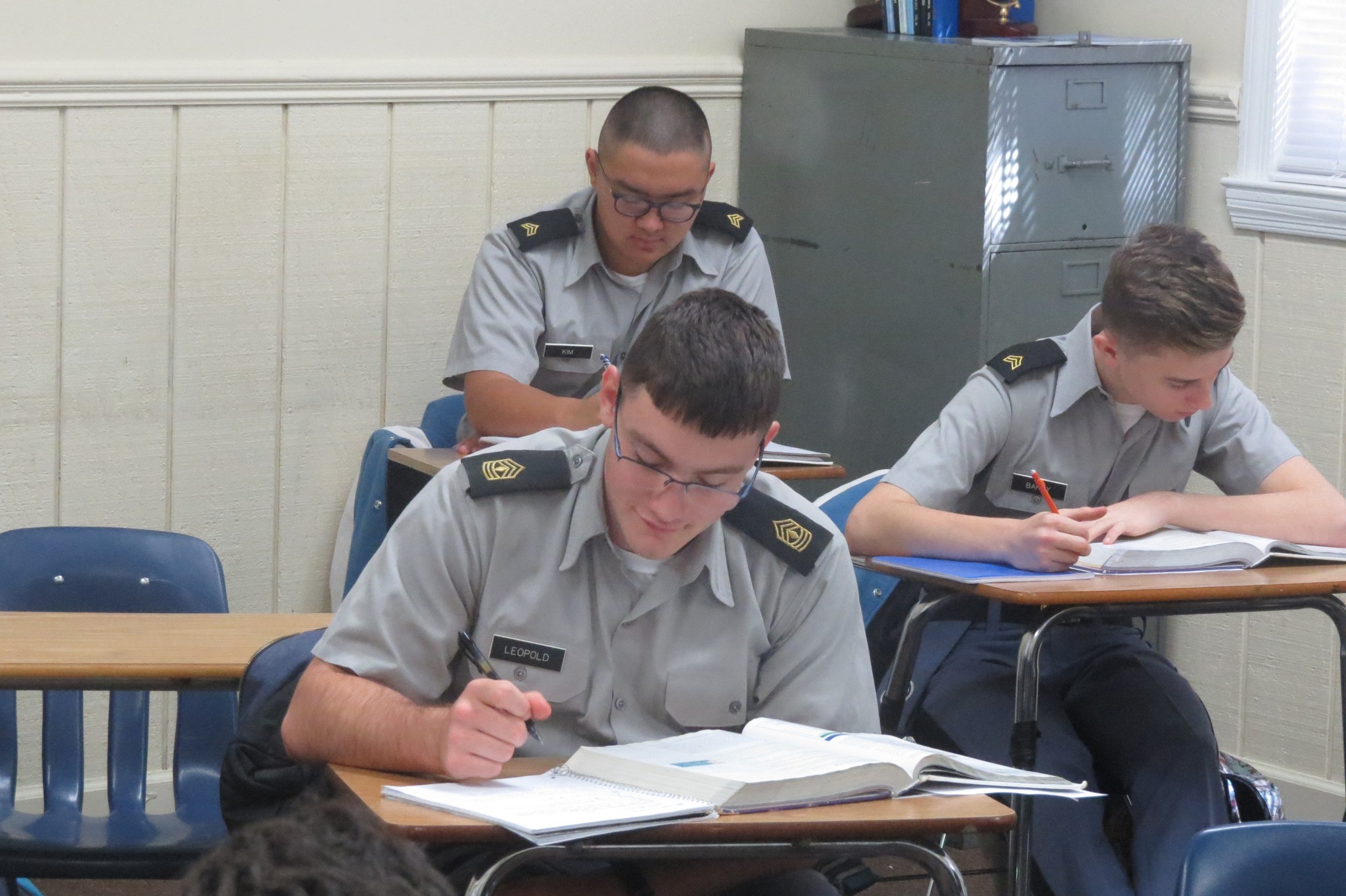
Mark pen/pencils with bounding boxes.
[600,353,612,370]
[456,630,544,746]
[1030,470,1061,514]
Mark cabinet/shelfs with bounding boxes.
[739,26,1191,499]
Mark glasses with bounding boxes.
[614,377,765,511]
[597,154,706,223]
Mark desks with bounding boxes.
[392,448,845,479]
[844,547,1346,896]
[0,611,340,691]
[325,750,1020,896]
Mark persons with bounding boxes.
[281,290,883,896]
[846,224,1346,896]
[176,799,457,896]
[442,87,791,455]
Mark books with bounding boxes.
[563,717,1107,816]
[380,763,719,848]
[1069,527,1346,573]
[479,436,833,466]
[872,556,1095,585]
[879,0,1033,38]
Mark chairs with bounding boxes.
[0,464,1345,896]
